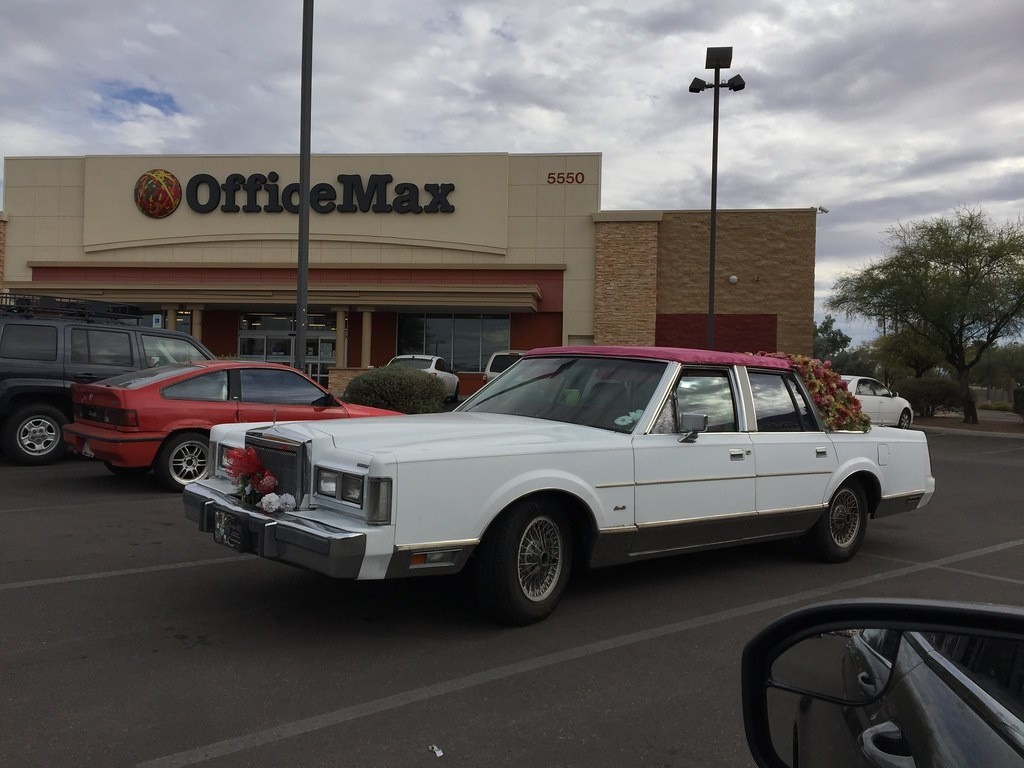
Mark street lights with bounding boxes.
[689,47,746,350]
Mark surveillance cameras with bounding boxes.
[816,206,829,214]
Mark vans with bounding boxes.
[483,351,528,389]
[0,306,221,464]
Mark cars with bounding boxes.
[791,375,912,429]
[61,358,407,492]
[183,345,937,623]
[387,354,460,402]
[843,626,1024,768]
